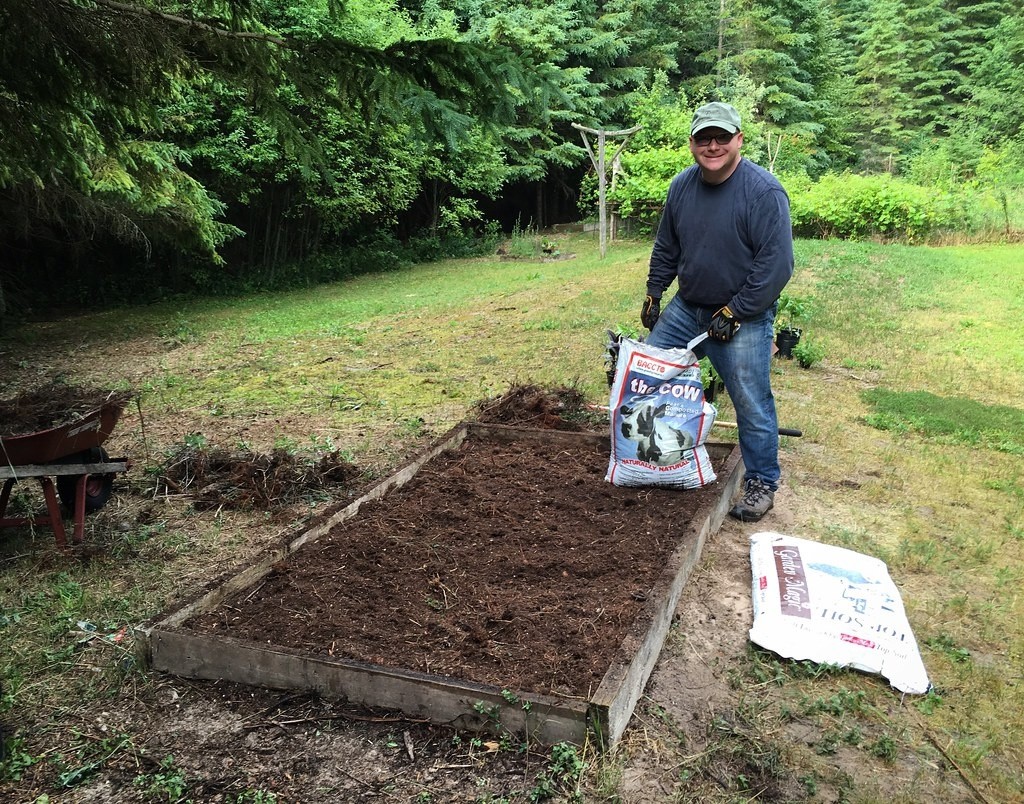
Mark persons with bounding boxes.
[636,101,795,523]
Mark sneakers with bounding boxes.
[729,476,774,522]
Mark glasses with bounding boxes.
[694,131,739,147]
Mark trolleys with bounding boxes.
[0,390,137,560]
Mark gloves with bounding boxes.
[641,294,660,331]
[708,306,739,345]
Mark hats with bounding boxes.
[691,102,741,136]
